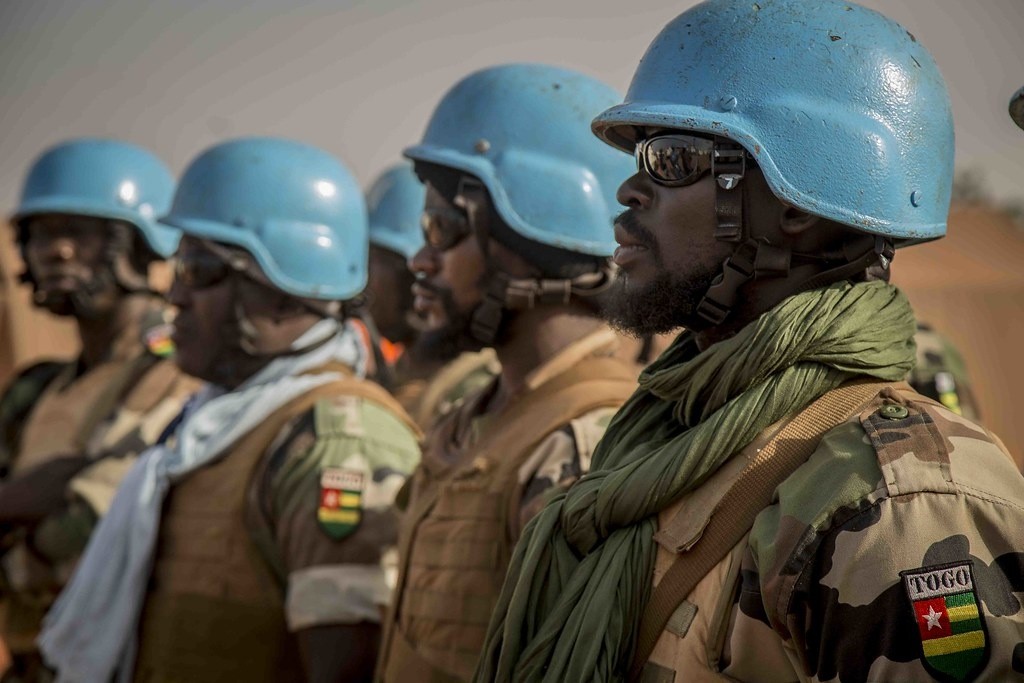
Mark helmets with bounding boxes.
[404,62,638,256]
[157,136,368,300]
[591,0,955,248]
[364,164,427,259]
[8,136,182,258]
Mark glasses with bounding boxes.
[635,130,753,186]
[174,234,248,286]
[420,171,491,249]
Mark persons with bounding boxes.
[0,131,212,683]
[470,0,1024,683]
[44,136,501,683]
[372,62,641,683]
